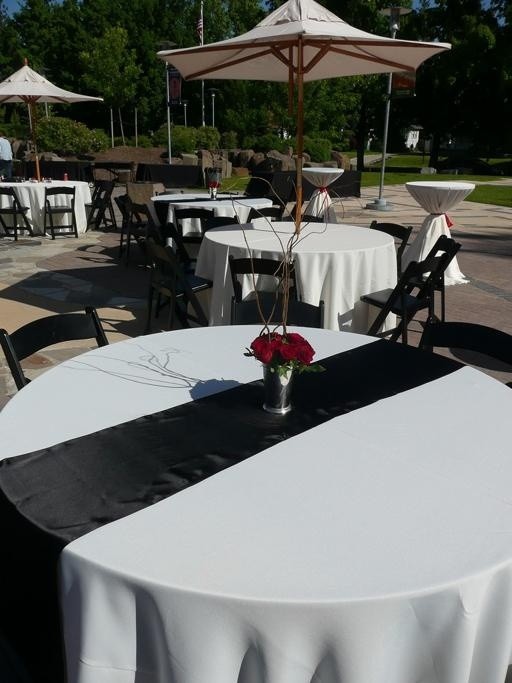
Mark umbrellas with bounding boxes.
[154,0,453,236]
[0,56,106,183]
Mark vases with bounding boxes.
[261,361,296,414]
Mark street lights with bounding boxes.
[376,4,412,201]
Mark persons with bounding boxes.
[0,129,14,179]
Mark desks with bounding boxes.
[11,160,92,183]
[250,170,361,203]
[0,322,512,683]
[136,163,203,188]
